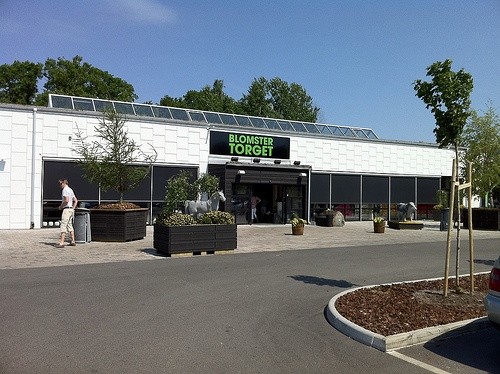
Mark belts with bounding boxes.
[64,207,72,209]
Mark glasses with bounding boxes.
[60,182,63,185]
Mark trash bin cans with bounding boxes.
[73,207,92,245]
[438,207,452,232]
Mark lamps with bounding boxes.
[237,170,245,174]
[299,173,307,177]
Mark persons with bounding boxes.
[251,193,262,224]
[53,178,78,248]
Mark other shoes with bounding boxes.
[67,243,76,246]
[55,245,64,248]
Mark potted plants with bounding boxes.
[290,213,307,235]
[154,169,237,253]
[372,213,385,233]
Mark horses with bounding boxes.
[396,202,417,222]
[185,190,226,215]
